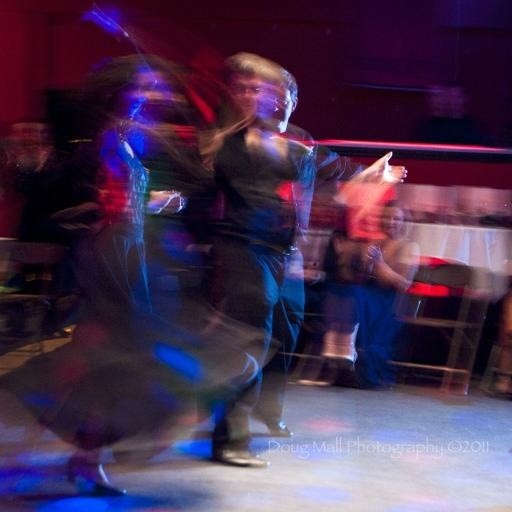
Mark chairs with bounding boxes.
[270,253,493,393]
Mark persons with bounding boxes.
[292,200,368,385]
[3,118,94,334]
[1,47,254,497]
[356,199,421,390]
[208,54,409,472]
